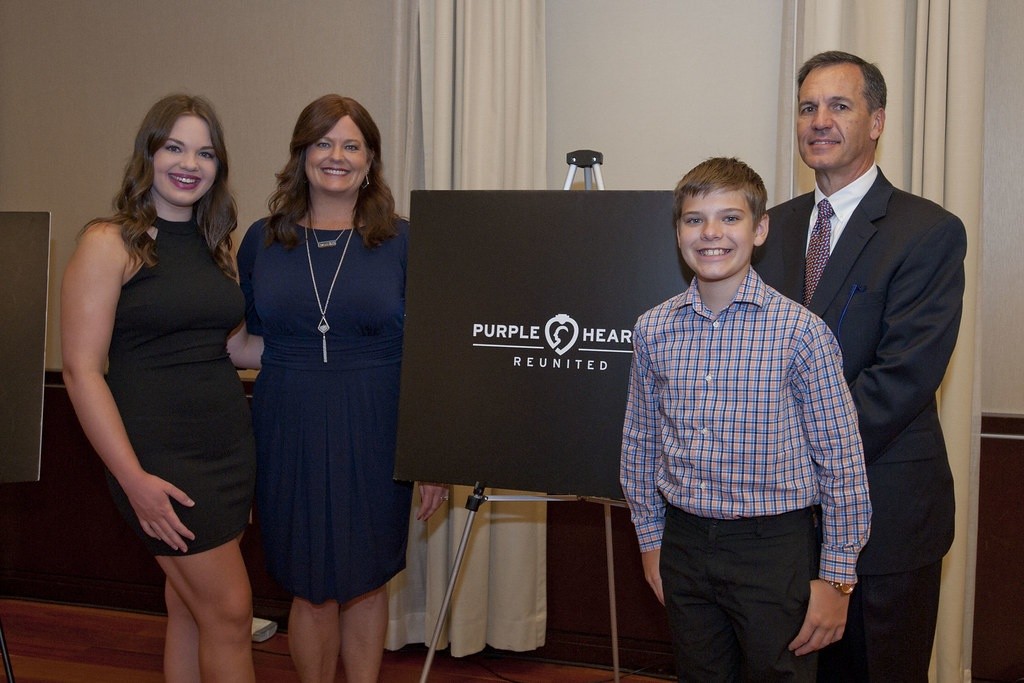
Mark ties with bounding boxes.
[802,201,834,315]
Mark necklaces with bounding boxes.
[309,221,347,249]
[303,210,354,364]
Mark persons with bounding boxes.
[59,95,454,683]
[621,157,873,683]
[748,51,969,683]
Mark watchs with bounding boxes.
[825,579,855,593]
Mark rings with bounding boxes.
[444,495,449,500]
[440,495,444,501]
